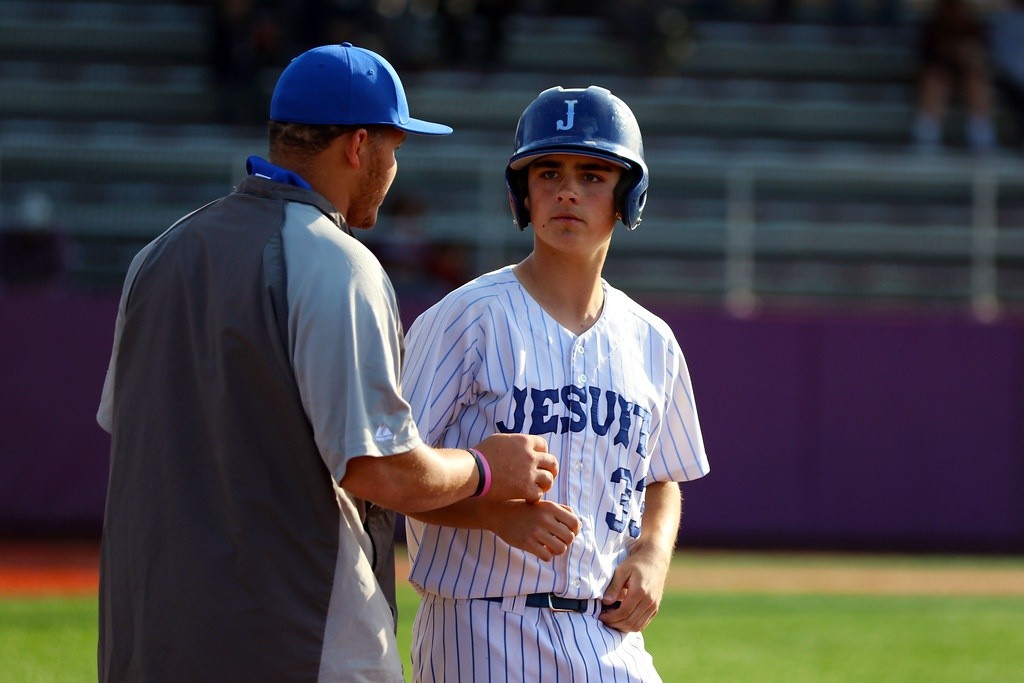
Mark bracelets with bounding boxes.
[465,448,491,497]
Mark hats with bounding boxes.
[270,41,454,136]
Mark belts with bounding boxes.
[474,590,622,616]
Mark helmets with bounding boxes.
[504,85,650,233]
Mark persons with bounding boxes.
[374,85,712,682]
[94,42,558,683]
